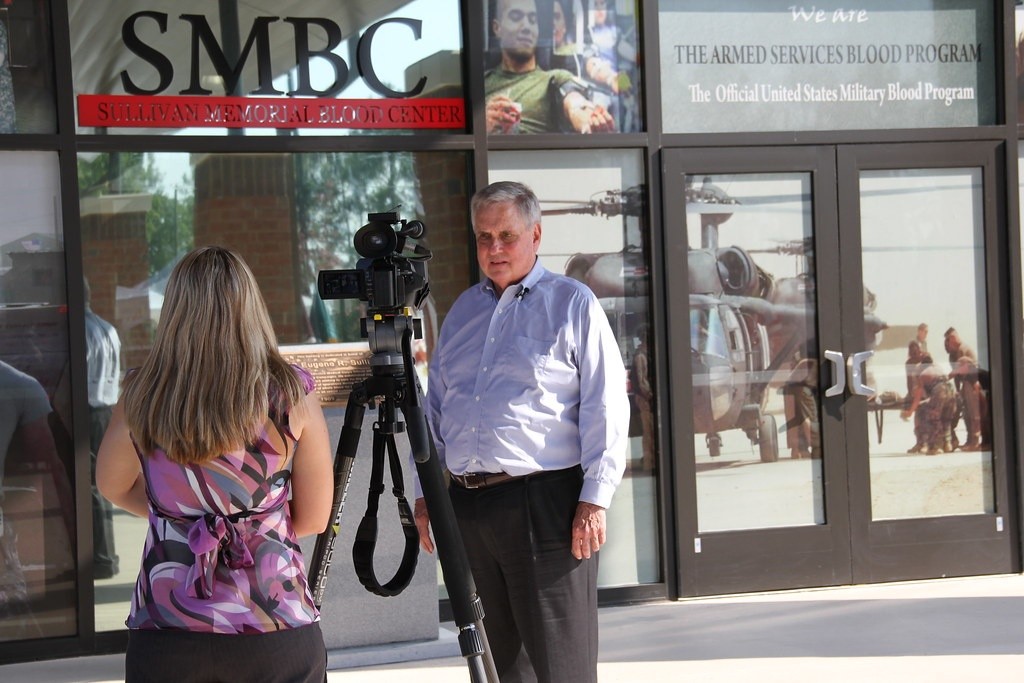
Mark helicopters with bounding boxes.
[529,175,978,463]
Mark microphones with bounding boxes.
[404,219,427,240]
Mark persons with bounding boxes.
[782,315,990,460]
[633,325,655,475]
[0,358,74,640]
[413,181,631,683]
[485,0,639,136]
[95,244,334,683]
[85,276,122,579]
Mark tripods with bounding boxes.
[304,307,501,683]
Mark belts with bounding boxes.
[450,472,513,488]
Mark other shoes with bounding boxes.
[907,439,992,455]
[57,554,119,578]
